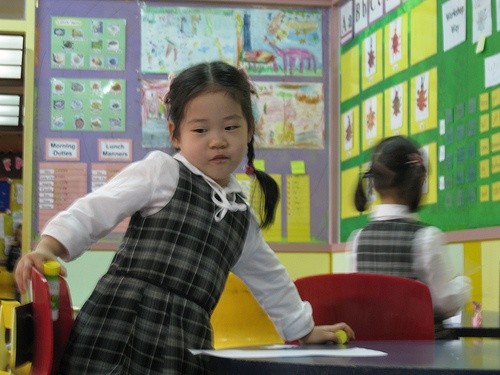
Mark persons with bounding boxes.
[14,62,355,375]
[347,137,475,338]
[6,225,23,273]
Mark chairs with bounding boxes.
[210,271,283,349]
[32,266,74,375]
[283,273,433,345]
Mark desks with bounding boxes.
[442,310,500,336]
[207,340,500,375]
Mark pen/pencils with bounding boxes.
[264,344,298,349]
